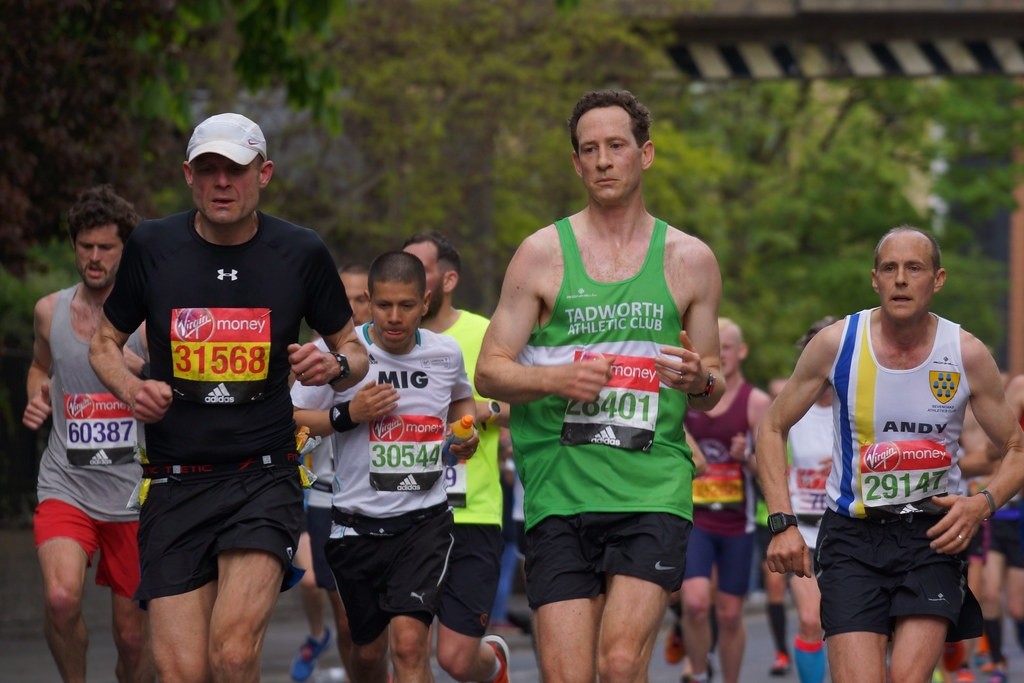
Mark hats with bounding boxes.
[187,114,267,165]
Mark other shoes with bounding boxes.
[933,636,1009,682]
[769,651,791,675]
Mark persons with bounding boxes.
[21,184,156,683]
[289,230,1024,683]
[754,224,1024,683]
[88,111,370,683]
[473,91,727,683]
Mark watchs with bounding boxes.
[686,371,717,401]
[328,352,350,386]
[481,400,501,431]
[767,512,798,537]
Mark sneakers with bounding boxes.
[291,626,331,683]
[482,635,511,683]
[664,622,685,665]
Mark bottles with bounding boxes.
[442,415,474,468]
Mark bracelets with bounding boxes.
[330,400,360,433]
[473,423,477,430]
[976,490,996,522]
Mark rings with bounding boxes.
[302,373,306,380]
[958,535,964,541]
